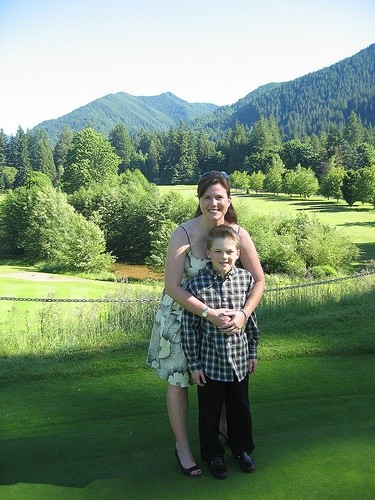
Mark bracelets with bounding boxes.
[241,309,249,323]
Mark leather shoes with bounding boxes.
[231,450,256,472]
[209,456,228,478]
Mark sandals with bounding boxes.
[174,448,202,476]
[219,430,228,442]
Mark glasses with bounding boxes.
[199,171,230,181]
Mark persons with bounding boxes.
[181,224,260,480]
[146,171,268,477]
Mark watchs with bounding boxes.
[201,306,211,319]
